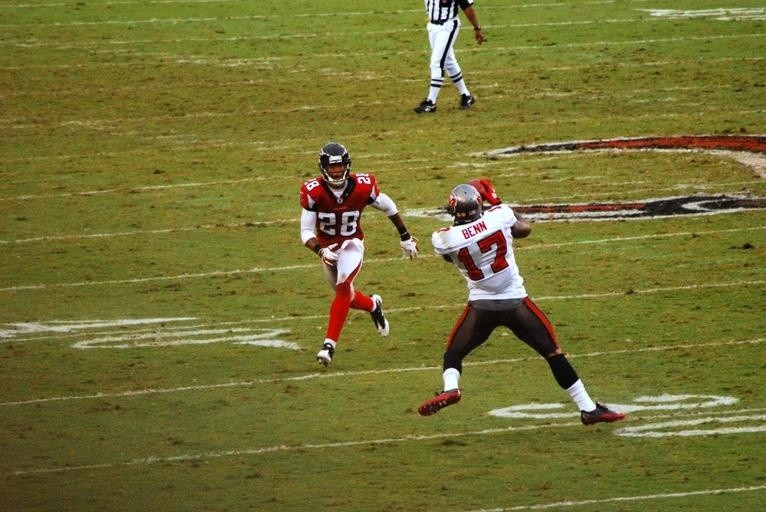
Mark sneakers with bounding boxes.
[414,99,436,113]
[317,343,335,367]
[581,401,625,425]
[370,294,389,337]
[418,389,461,416]
[460,93,475,110]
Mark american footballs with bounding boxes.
[467,181,486,201]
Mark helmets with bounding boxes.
[318,143,350,188]
[447,183,484,225]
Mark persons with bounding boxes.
[416,177,625,427]
[298,144,420,368]
[413,0,484,115]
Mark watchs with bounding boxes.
[473,25,482,32]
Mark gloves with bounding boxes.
[317,243,339,267]
[478,179,502,205]
[400,235,419,261]
